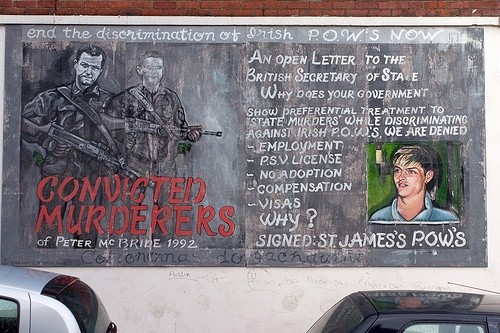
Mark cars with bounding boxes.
[305,290,500,333]
[0,263,117,333]
